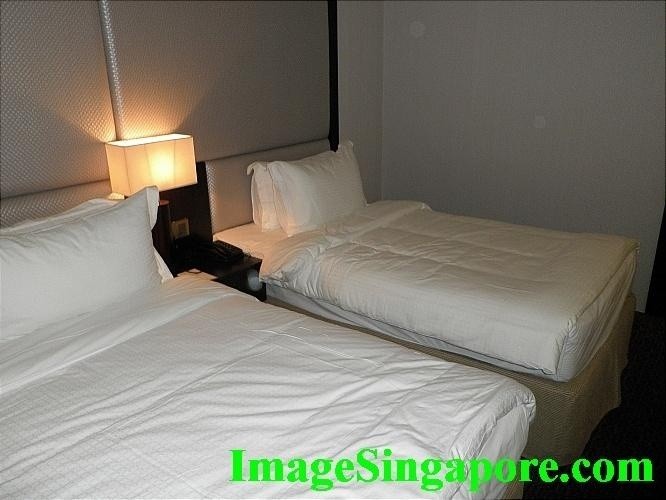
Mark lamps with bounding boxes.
[105,133,198,265]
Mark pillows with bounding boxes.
[244,139,368,242]
[0,185,177,349]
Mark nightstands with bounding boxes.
[166,253,269,302]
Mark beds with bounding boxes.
[0,276,535,500]
[205,135,641,470]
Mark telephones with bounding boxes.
[210,240,244,263]
